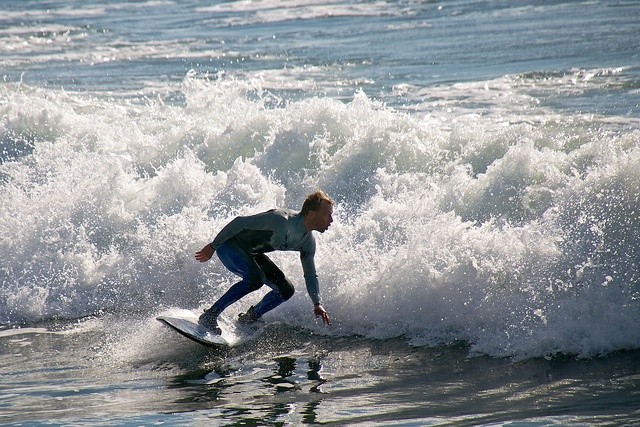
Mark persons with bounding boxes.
[194,190,335,335]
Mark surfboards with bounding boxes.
[156,312,256,348]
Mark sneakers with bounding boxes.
[238,312,265,328]
[198,309,222,335]
